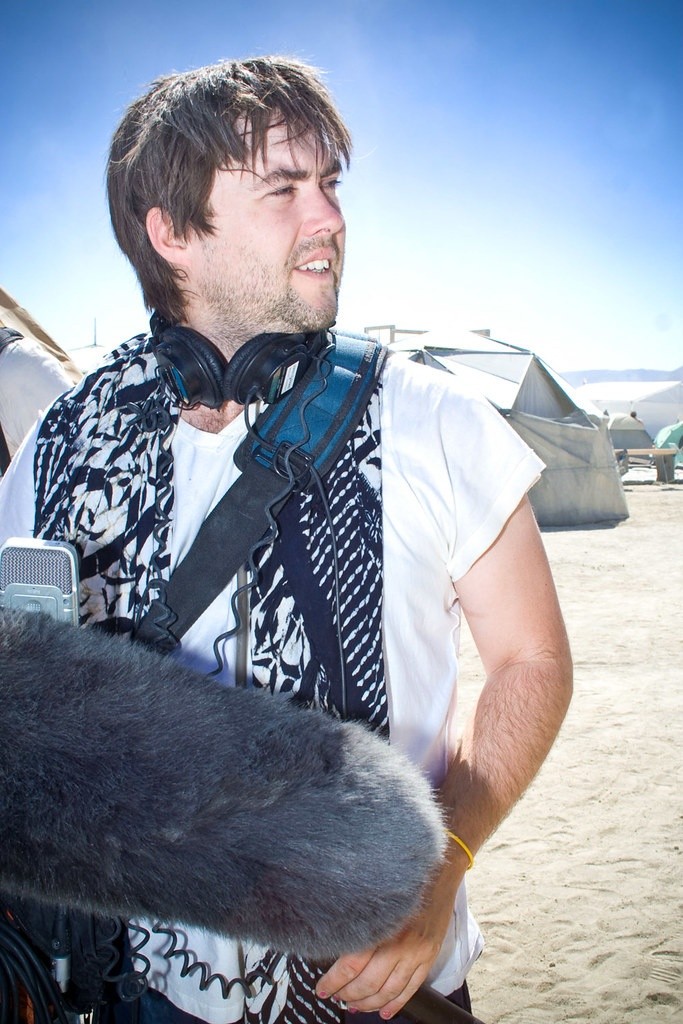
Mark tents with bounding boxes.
[377,328,683,527]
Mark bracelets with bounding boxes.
[438,827,475,870]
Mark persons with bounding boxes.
[0,57,574,1024]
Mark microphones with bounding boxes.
[0,537,81,629]
[0,607,448,958]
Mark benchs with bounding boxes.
[634,448,677,478]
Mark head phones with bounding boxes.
[149,311,331,412]
